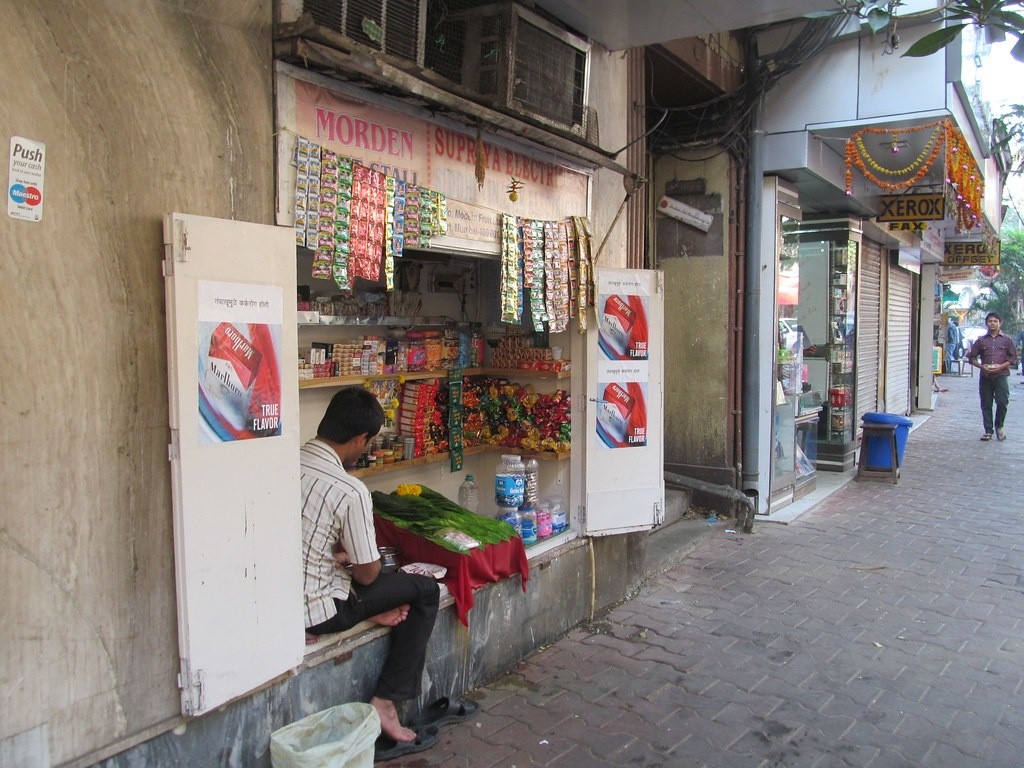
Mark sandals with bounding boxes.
[981,432,992,441]
[995,425,1006,441]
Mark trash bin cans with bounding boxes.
[269,702,382,768]
[860,412,913,475]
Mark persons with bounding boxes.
[1012,330,1024,385]
[300,385,440,742]
[966,312,1018,441]
[933,372,948,392]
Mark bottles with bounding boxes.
[358,434,414,467]
[458,474,478,513]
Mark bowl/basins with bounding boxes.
[378,547,402,572]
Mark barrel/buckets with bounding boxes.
[860,411,912,471]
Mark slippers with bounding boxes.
[412,695,480,727]
[374,725,442,760]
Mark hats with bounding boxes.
[953,321,959,326]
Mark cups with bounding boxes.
[552,346,562,361]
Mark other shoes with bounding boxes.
[1020,381,1024,384]
[937,387,948,392]
